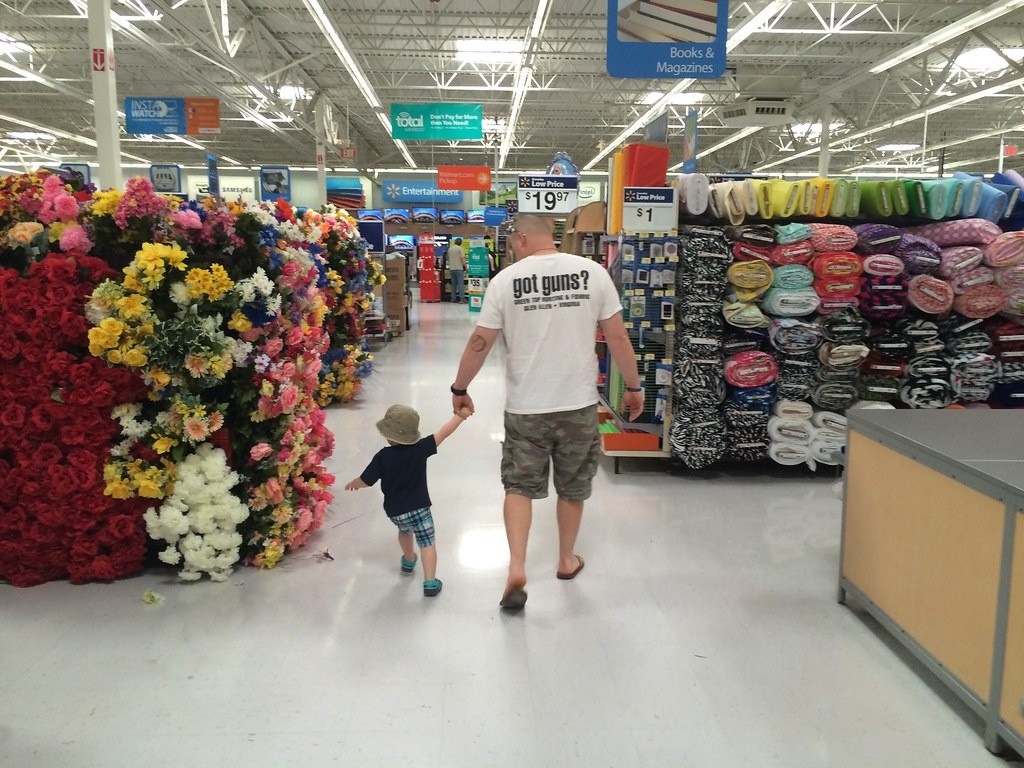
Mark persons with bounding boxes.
[486,243,499,280]
[449,214,645,611]
[445,238,467,304]
[344,404,469,596]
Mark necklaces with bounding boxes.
[529,248,560,256]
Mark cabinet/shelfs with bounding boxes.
[559,200,607,268]
[837,408,1024,761]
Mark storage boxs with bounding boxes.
[596,410,660,454]
[365,238,492,336]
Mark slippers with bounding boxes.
[500,589,528,610]
[557,552,585,580]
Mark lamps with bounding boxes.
[0,0,1024,194]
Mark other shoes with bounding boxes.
[422,578,442,597]
[401,554,418,573]
[459,300,468,304]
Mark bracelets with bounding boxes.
[624,387,642,392]
[451,383,467,396]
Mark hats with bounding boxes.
[375,402,421,445]
